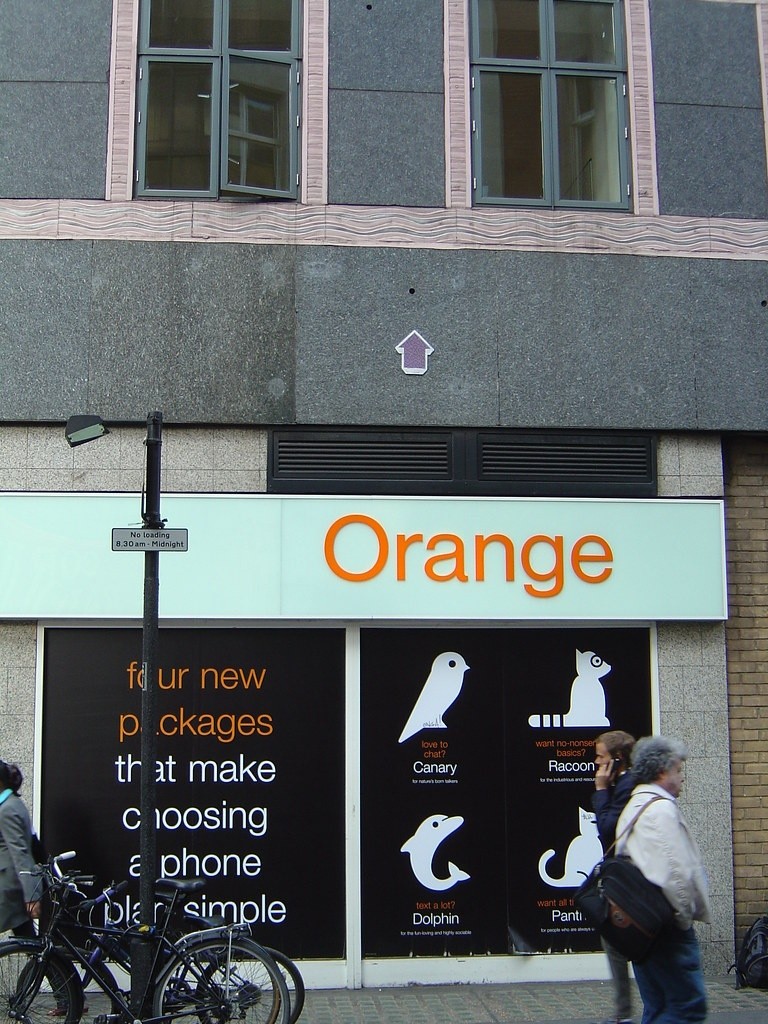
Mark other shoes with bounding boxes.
[47,1006,89,1017]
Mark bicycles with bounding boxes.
[0,850,304,1024]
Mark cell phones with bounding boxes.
[606,757,621,774]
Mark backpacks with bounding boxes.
[733,915,768,990]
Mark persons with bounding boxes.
[592,731,636,1024]
[0,758,89,1017]
[615,736,714,1024]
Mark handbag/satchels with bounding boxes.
[30,833,48,864]
[573,856,680,960]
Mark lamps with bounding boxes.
[65,416,110,448]
